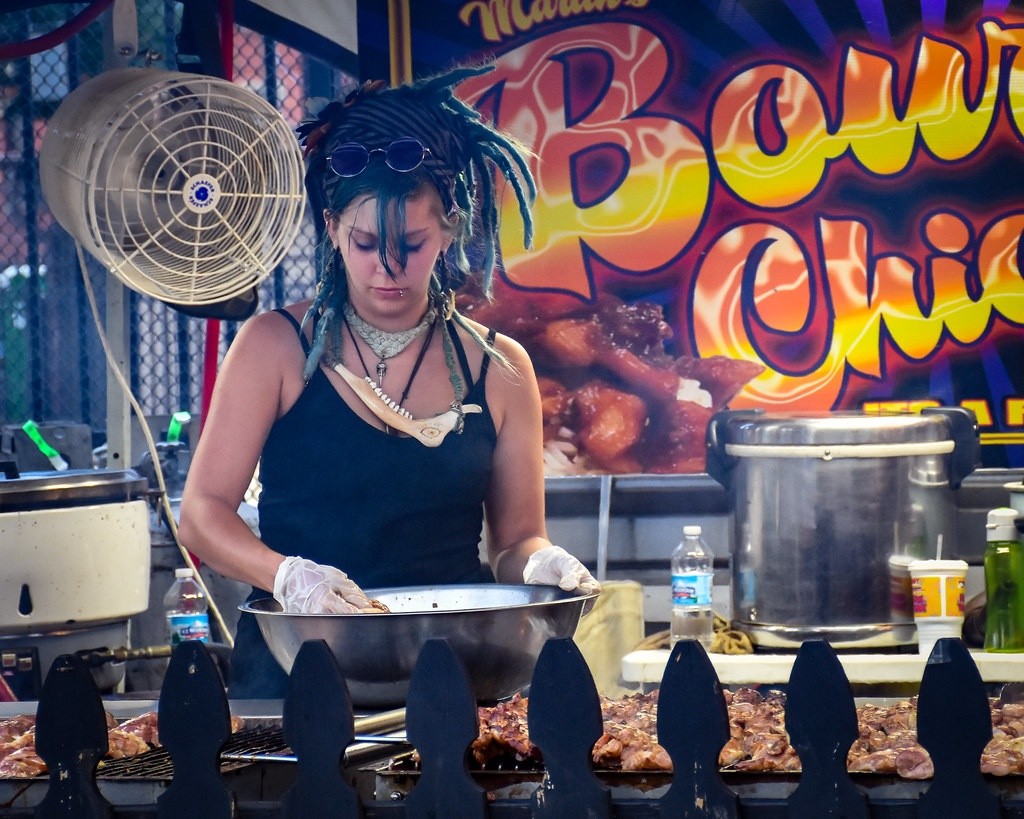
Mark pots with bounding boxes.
[704,403,981,651]
[0,468,163,638]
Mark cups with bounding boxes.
[908,558,969,658]
[890,554,916,640]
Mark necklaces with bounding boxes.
[342,289,437,438]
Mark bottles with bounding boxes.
[162,568,210,646]
[669,525,715,652]
[983,506,1024,652]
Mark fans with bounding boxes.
[36,65,308,307]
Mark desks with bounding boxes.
[618,649,1024,684]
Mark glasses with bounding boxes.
[324,136,453,189]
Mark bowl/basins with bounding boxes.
[237,582,601,702]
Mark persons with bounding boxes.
[179,65,602,701]
